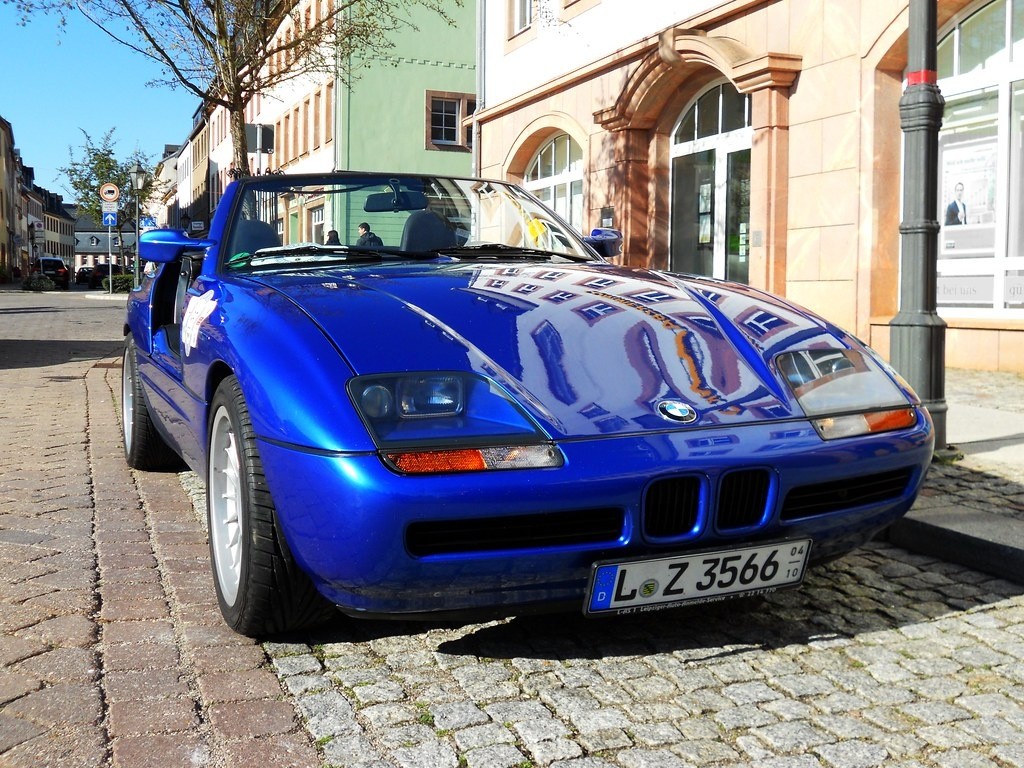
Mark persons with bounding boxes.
[945,180,968,225]
[356,221,383,249]
[325,231,343,246]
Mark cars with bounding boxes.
[76,266,95,286]
[29,257,70,291]
[95,263,122,288]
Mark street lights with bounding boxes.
[127,159,147,287]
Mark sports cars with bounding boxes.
[121,170,937,637]
